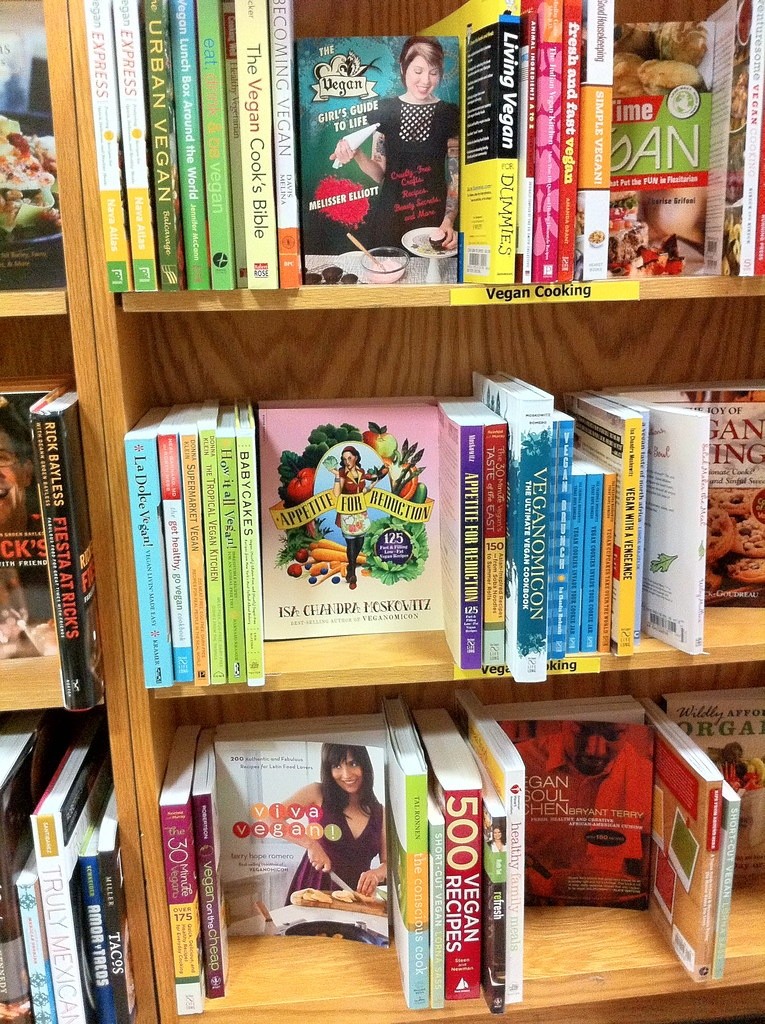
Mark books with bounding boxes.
[0,0,66,292]
[158,687,765,1015]
[0,721,138,1024]
[83,0,765,293]
[123,372,765,687]
[0,378,104,710]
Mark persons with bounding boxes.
[329,37,460,258]
[516,719,654,900]
[491,825,507,852]
[0,396,55,639]
[270,742,388,907]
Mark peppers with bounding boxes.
[379,449,418,502]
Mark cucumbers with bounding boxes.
[410,483,428,504]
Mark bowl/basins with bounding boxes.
[361,246,410,284]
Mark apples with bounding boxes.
[361,431,397,457]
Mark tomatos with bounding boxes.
[287,467,316,505]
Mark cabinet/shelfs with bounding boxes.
[68,0,765,1024]
[0,0,158,1024]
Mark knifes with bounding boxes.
[328,870,354,894]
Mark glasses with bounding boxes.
[0,449,26,468]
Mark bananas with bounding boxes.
[738,756,765,785]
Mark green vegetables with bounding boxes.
[361,514,430,585]
[277,423,363,509]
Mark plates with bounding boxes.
[401,226,458,259]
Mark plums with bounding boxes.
[287,549,308,577]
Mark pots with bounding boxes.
[285,921,381,945]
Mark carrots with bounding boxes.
[309,538,368,576]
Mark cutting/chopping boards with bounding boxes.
[301,890,387,914]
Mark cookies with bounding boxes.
[703,488,765,598]
